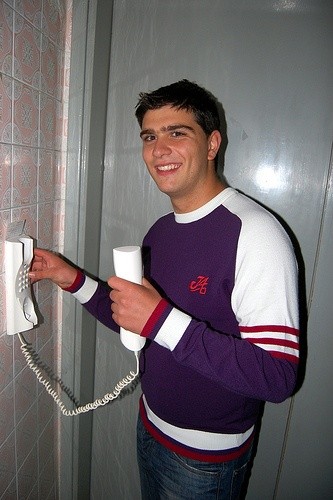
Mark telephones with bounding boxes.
[110,245,147,353]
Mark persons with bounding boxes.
[28,79,304,500]
